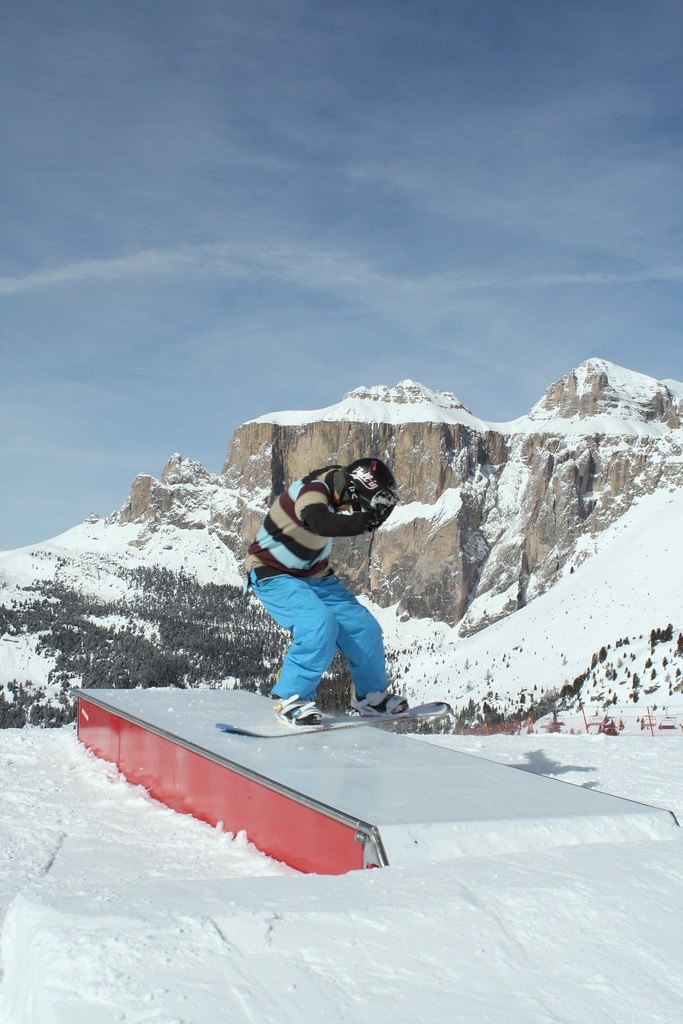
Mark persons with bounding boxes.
[244,459,409,727]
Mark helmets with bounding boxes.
[344,458,395,512]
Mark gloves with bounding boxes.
[371,488,400,521]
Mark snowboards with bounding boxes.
[215,701,453,740]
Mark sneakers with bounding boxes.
[272,696,323,727]
[349,683,409,717]
